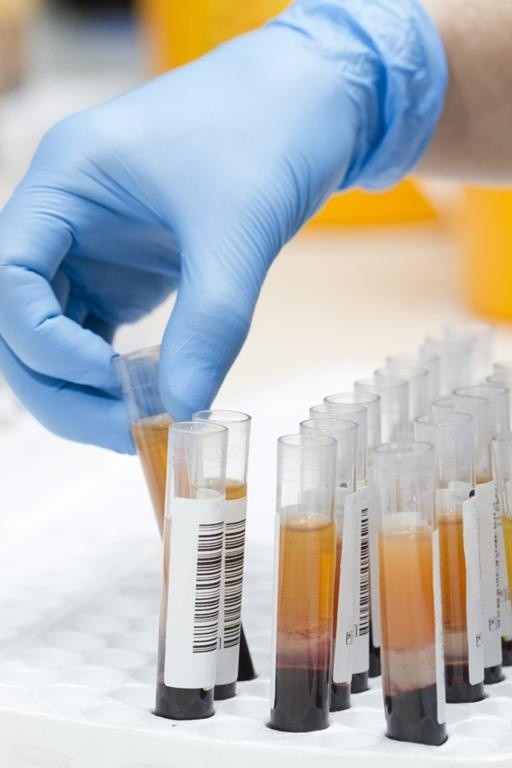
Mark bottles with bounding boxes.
[112,343,258,723]
[263,321,512,745]
